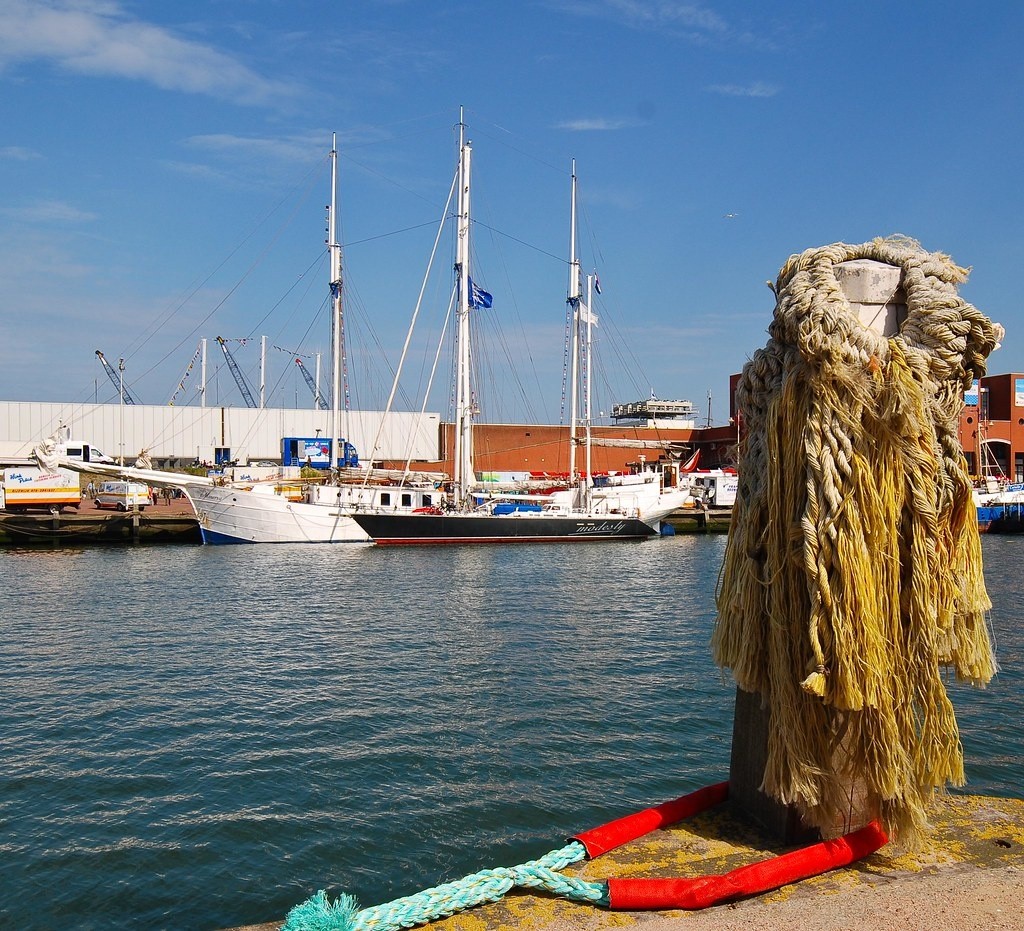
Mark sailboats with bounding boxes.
[959,407,1024,532]
[350,104,691,546]
[41,130,660,546]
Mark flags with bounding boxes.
[446,311,479,425]
[167,337,317,412]
[594,271,604,296]
[324,204,350,411]
[457,278,493,312]
[559,300,596,428]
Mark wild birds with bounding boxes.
[722,213,739,218]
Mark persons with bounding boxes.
[81,476,184,508]
[186,455,218,470]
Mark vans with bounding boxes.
[94,480,149,511]
[280,437,358,468]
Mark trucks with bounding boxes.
[0,440,114,468]
[0,468,81,514]
